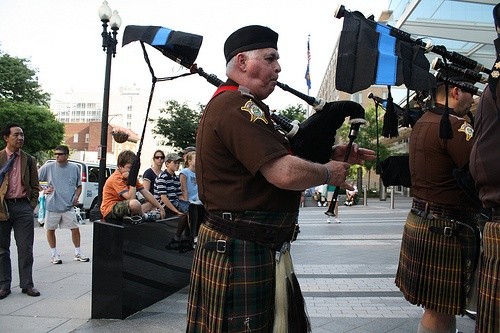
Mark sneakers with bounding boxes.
[51,252,62,264]
[74,251,89,262]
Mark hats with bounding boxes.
[223,26,278,61]
[436,64,475,86]
[165,153,182,163]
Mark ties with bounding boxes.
[0,152,17,176]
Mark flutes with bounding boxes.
[124,25,366,216]
[333,5,500,128]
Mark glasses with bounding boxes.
[154,156,164,160]
[54,153,66,156]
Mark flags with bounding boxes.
[305,38,311,89]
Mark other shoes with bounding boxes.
[21,288,40,297]
[122,215,143,225]
[165,238,183,250]
[142,213,159,221]
[0,289,11,299]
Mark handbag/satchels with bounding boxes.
[38,191,46,224]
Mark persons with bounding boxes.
[0,2,500,333]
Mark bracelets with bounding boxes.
[323,165,331,185]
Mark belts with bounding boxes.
[204,213,298,242]
[412,201,477,221]
[4,197,27,203]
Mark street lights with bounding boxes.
[90,0,123,221]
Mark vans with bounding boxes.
[38,158,118,219]
